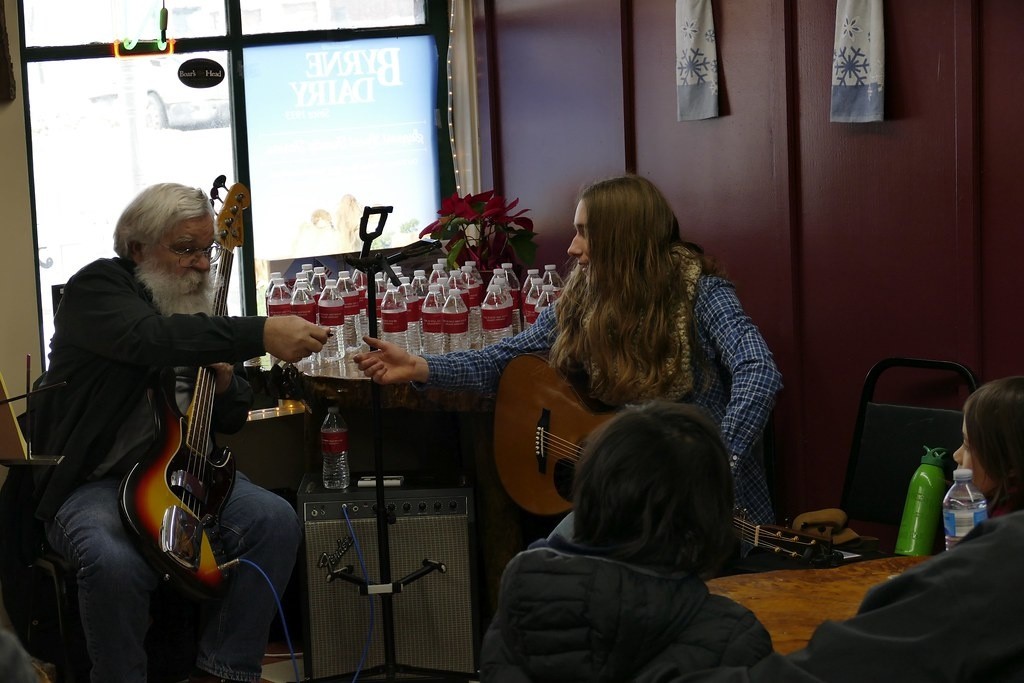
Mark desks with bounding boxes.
[705,556,924,656]
[257,359,602,616]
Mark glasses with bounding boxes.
[158,243,218,268]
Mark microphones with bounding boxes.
[325,564,354,583]
[421,559,447,573]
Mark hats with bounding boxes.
[791,508,859,546]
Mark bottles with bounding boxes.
[894,446,948,556]
[942,468,989,551]
[265,258,565,367]
[320,407,350,489]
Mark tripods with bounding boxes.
[304,205,480,683]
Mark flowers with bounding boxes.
[418,189,540,271]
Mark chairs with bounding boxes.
[838,357,981,539]
[24,370,210,683]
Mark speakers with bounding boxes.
[296,470,481,683]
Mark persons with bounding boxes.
[480,401,773,683]
[952,375,1023,517]
[31,184,331,683]
[629,510,1024,683]
[353,176,784,576]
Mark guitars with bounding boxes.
[493,348,833,569]
[119,175,253,590]
